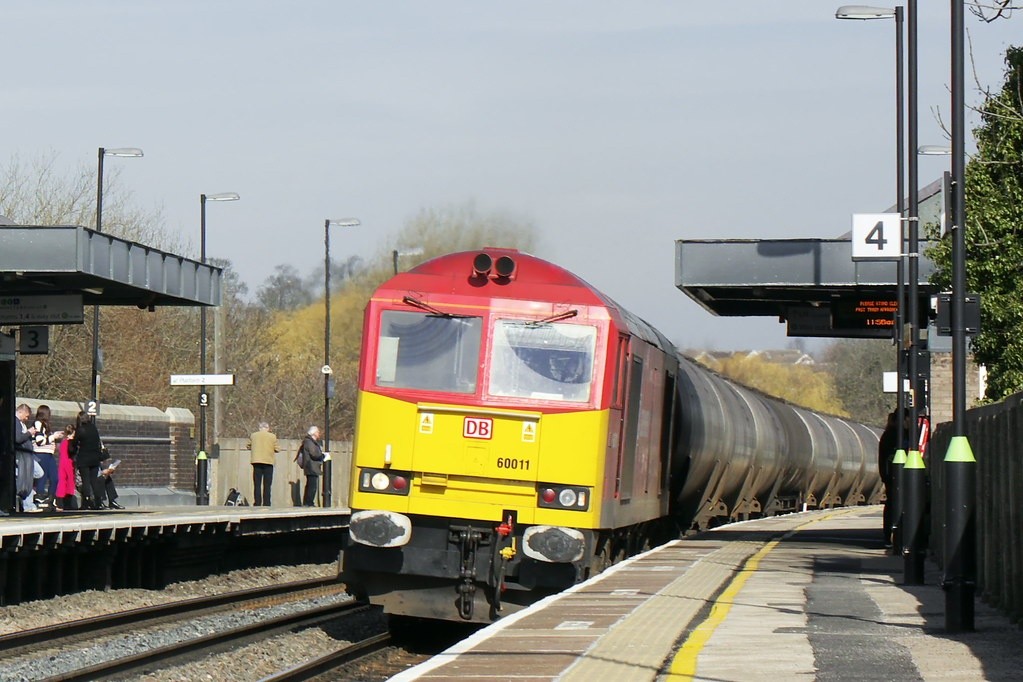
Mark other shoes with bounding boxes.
[23,506,43,513]
[52,505,63,512]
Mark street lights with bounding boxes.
[392,245,424,275]
[196,191,242,506]
[322,217,362,509]
[91,147,145,425]
[833,3,906,556]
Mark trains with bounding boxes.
[339,246,888,639]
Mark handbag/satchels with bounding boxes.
[32,442,55,454]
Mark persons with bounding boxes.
[879,407,910,547]
[303,425,326,507]
[31,404,125,511]
[247,422,280,506]
[0,395,11,516]
[15,405,44,514]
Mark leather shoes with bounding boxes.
[108,501,126,509]
[101,502,111,509]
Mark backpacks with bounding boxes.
[293,443,305,469]
